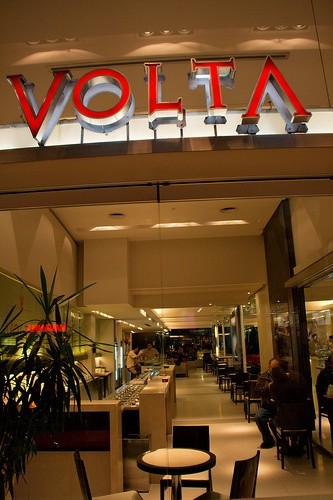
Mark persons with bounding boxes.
[255,358,281,449]
[138,342,159,362]
[268,371,309,457]
[127,345,141,377]
[308,334,322,356]
[315,353,333,444]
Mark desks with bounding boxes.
[137,446,216,500]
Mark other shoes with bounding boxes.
[260,440,275,448]
[279,443,306,458]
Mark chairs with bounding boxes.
[73,449,144,500]
[193,450,260,500]
[160,426,213,500]
[203,354,333,470]
